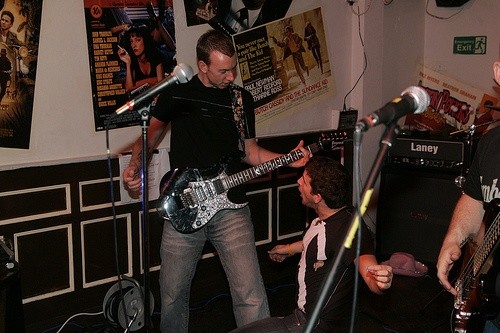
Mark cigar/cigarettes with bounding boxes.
[118,45,128,54]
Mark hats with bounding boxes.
[380,251,428,277]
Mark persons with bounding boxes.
[117,26,164,92]
[0,49,13,103]
[436,60,500,295]
[123,31,313,333]
[103,7,133,51]
[268,156,393,333]
[269,27,309,86]
[304,21,323,74]
[0,11,22,51]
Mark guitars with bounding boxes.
[159,127,353,234]
[449,211,499,333]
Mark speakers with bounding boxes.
[375,163,471,333]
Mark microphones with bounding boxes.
[355,86,431,132]
[111,63,193,119]
